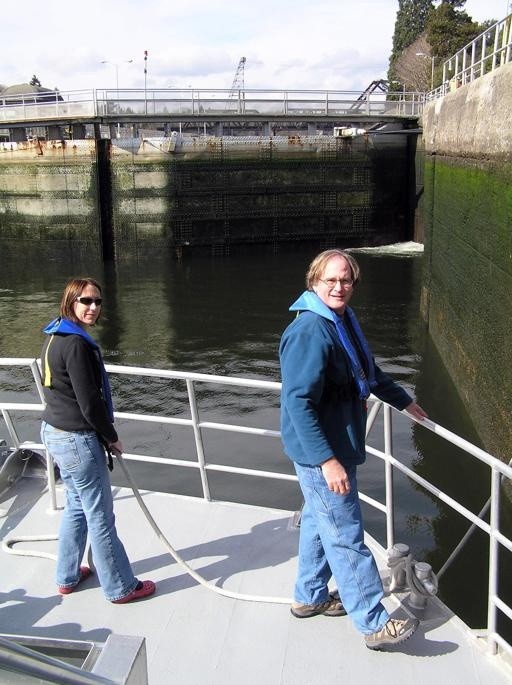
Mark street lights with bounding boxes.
[414,51,435,90]
[99,58,134,137]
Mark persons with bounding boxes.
[275,248,430,652]
[39,276,157,605]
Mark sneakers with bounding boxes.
[365,618,419,650]
[291,590,347,618]
[111,581,155,603]
[59,566,89,594]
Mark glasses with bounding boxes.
[77,297,102,305]
[319,279,353,288]
